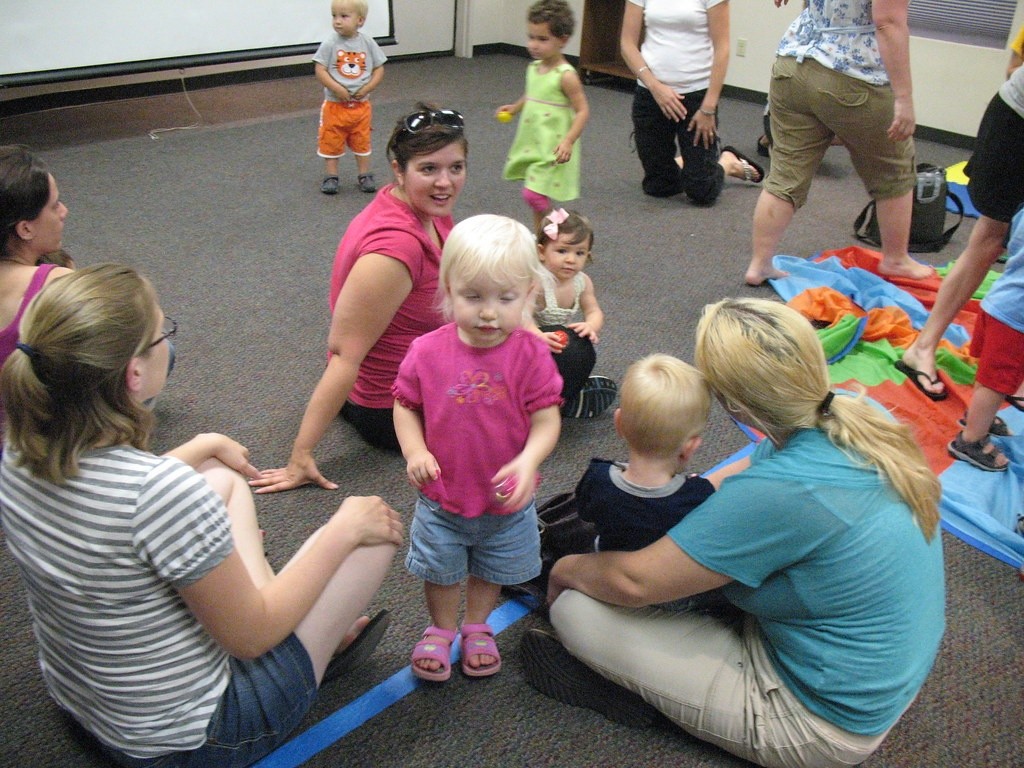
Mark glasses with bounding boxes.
[148,315,178,347]
[396,108,464,144]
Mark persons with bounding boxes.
[1,144,174,414]
[312,0,387,195]
[0,264,404,768]
[744,0,934,286]
[521,208,604,354]
[894,26,1024,473]
[516,296,949,768]
[572,353,751,614]
[388,212,563,680]
[620,0,765,208]
[491,0,590,228]
[249,101,617,496]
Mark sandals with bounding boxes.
[412,622,458,681]
[320,609,390,689]
[462,619,501,677]
[947,429,1009,471]
[957,409,1013,437]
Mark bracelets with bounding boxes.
[636,66,650,79]
[700,106,717,116]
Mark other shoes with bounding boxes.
[320,174,340,194]
[521,628,658,731]
[757,135,772,157]
[358,171,378,192]
[559,376,617,421]
[1015,513,1024,538]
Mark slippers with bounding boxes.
[722,146,764,184]
[894,360,947,401]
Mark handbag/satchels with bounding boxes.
[854,161,965,254]
[500,493,597,609]
